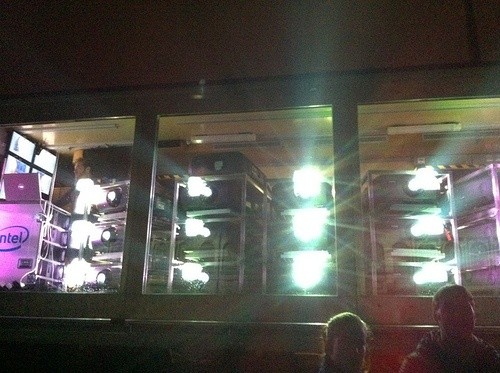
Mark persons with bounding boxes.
[399,286,500,373]
[319,312,374,373]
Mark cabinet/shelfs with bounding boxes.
[0,130,500,292]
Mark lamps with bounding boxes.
[387,123,462,135]
[191,133,256,144]
